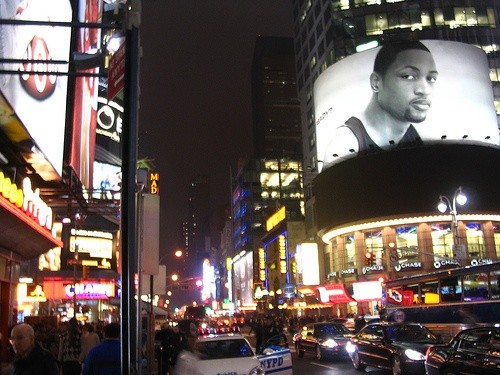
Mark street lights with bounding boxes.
[436,186,468,266]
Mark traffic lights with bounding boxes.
[365,252,372,259]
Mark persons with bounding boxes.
[9,323,57,375]
[0,293,327,375]
[320,39,439,171]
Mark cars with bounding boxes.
[173,332,293,375]
[424,326,500,375]
[346,320,443,375]
[291,321,355,361]
